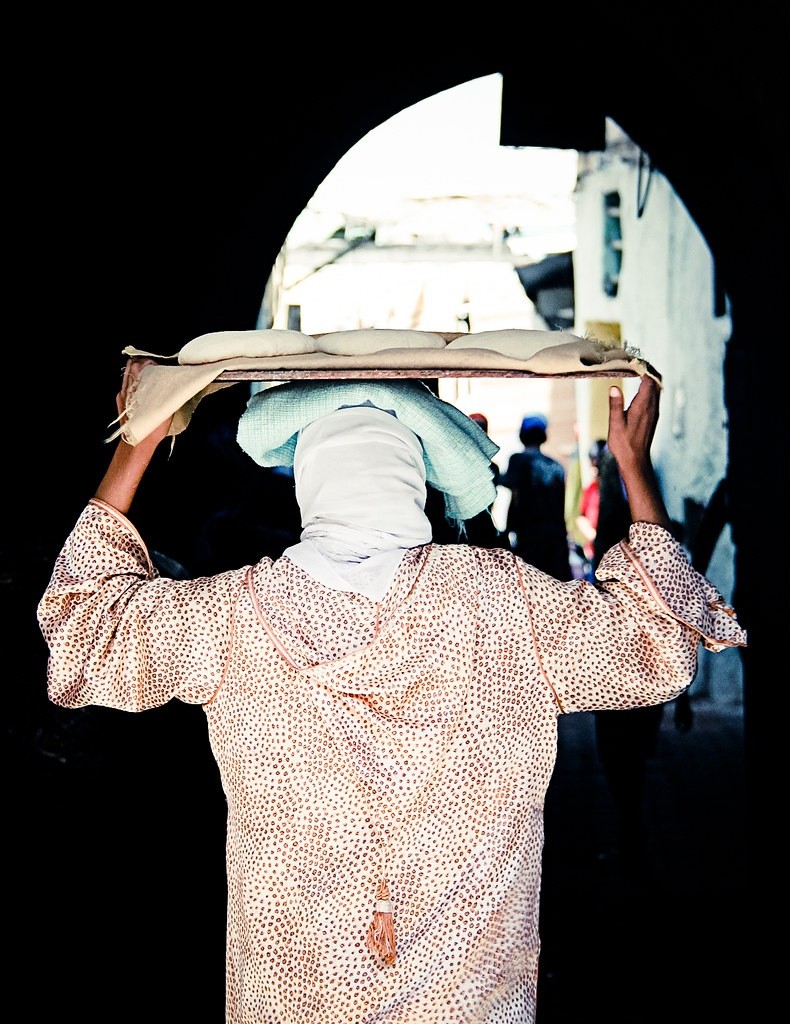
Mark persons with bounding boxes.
[591,452,663,736]
[499,412,577,582]
[36,355,749,1023]
[576,439,608,584]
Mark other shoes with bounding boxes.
[675,694,694,733]
